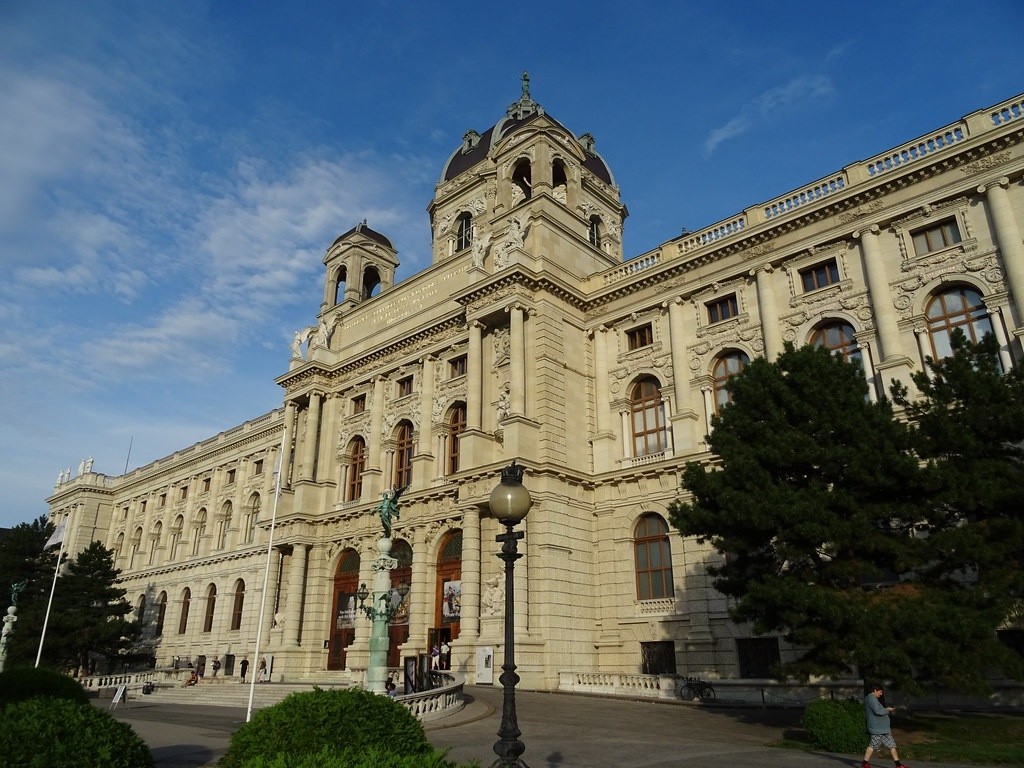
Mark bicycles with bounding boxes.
[680,677,717,703]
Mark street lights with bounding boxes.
[487,457,542,768]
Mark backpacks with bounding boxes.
[217,661,221,670]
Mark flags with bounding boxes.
[272,440,285,501]
[43,510,71,550]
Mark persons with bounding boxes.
[209,656,221,684]
[240,656,249,684]
[370,493,399,538]
[257,657,266,683]
[389,683,398,698]
[292,331,304,360]
[315,319,328,347]
[496,392,510,430]
[431,642,449,670]
[11,583,20,606]
[56,456,94,486]
[861,686,910,768]
[504,217,523,246]
[471,237,484,268]
[181,663,198,688]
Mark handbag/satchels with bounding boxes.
[264,667,267,675]
